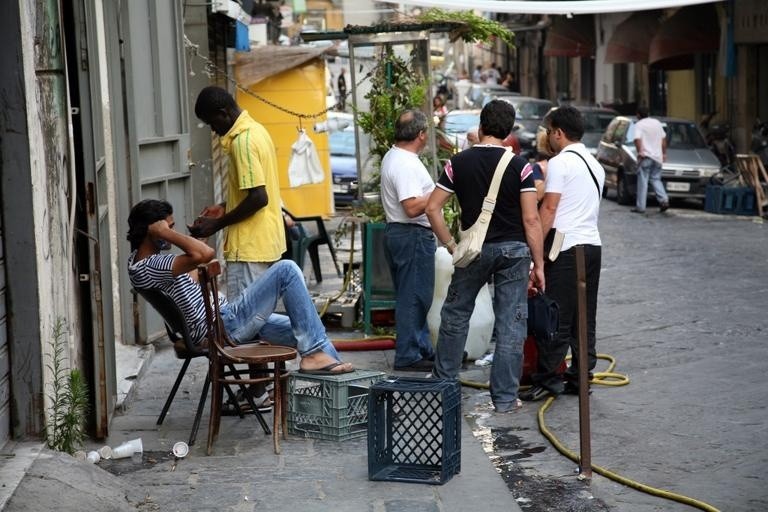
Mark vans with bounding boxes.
[429,46,447,72]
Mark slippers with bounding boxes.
[299,360,354,374]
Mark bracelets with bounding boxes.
[444,238,455,248]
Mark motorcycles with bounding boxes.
[752,113,767,155]
[435,70,454,101]
[698,107,735,170]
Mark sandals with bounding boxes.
[221,390,272,417]
[237,383,274,404]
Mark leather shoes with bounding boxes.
[660,202,669,211]
[631,208,644,213]
[393,360,433,372]
[428,351,467,364]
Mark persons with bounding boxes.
[339,67,347,111]
[519,104,605,403]
[520,129,568,386]
[279,203,310,240]
[474,62,519,93]
[425,100,547,414]
[433,90,454,129]
[474,134,546,367]
[380,111,448,372]
[186,88,285,414]
[127,198,355,374]
[631,105,669,213]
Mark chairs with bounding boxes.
[198,258,299,455]
[129,285,273,446]
[281,208,341,283]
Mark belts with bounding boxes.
[390,222,432,231]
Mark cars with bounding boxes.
[542,106,621,159]
[440,109,520,162]
[493,96,555,154]
[472,92,522,108]
[289,26,382,67]
[594,115,724,205]
[324,108,378,206]
[462,84,510,109]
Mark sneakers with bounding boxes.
[520,385,552,400]
[564,384,592,396]
[495,398,523,413]
[474,353,494,366]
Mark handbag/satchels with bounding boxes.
[451,211,491,268]
[528,287,560,349]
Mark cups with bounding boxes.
[86,438,143,464]
[173,441,189,460]
